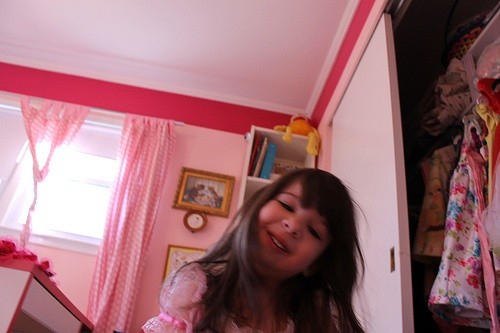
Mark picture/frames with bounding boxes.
[171,166,236,218]
[162,244,209,284]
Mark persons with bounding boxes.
[141,167,366,333]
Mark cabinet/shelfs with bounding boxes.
[236,124,316,211]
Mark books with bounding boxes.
[247,132,279,180]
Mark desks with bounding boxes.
[0,259,94,333]
